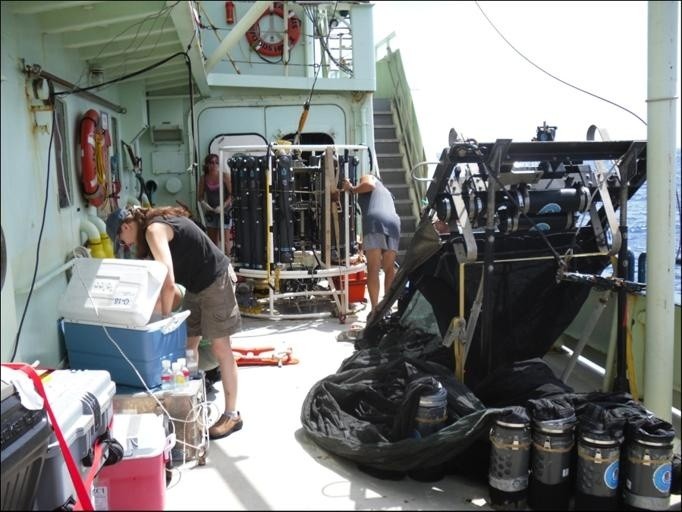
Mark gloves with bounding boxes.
[214,202,226,214]
[201,200,214,211]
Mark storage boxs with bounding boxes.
[56,253,208,512]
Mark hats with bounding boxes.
[106,208,128,251]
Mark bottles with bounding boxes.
[160,349,197,394]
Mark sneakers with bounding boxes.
[209,412,242,439]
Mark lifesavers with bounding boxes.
[245,1,301,56]
[77,108,110,207]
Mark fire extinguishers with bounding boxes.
[226,1,234,24]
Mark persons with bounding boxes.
[197,151,234,255]
[340,173,401,309]
[105,203,244,441]
